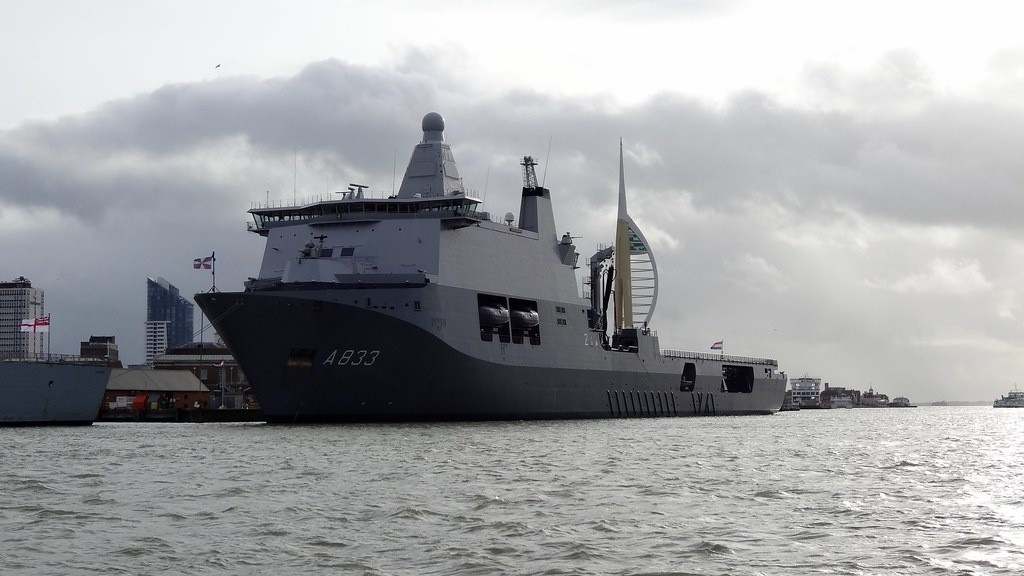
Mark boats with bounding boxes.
[889,397,917,407]
[993,383,1024,408]
[0,311,113,426]
[193,111,788,424]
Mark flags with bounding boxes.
[193,257,213,269]
[21,316,50,332]
[711,341,722,349]
